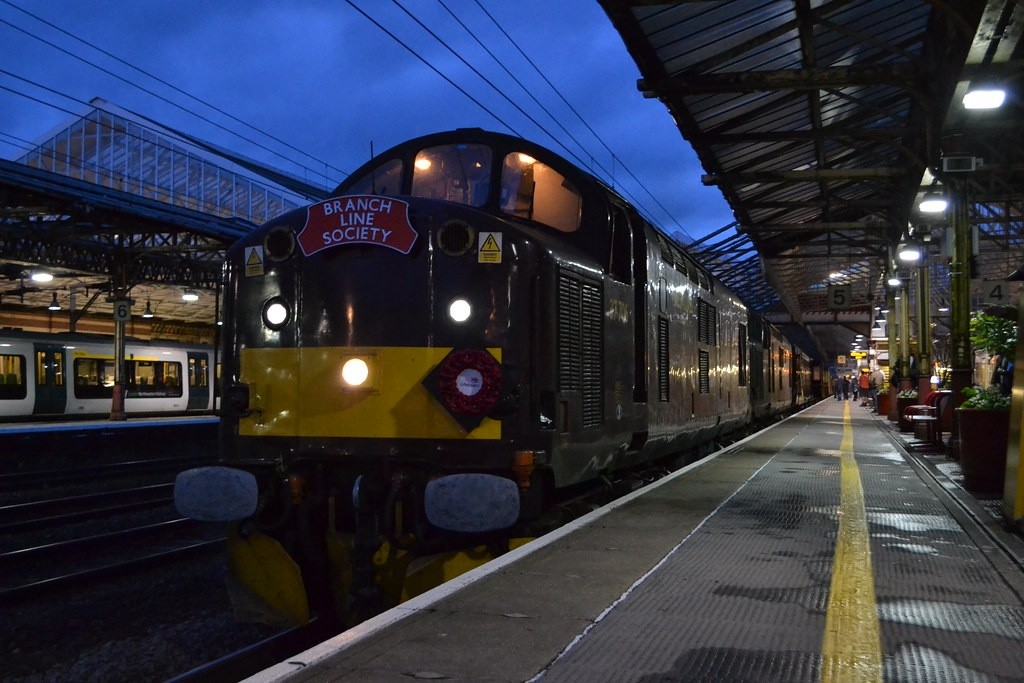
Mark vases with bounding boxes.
[877,395,890,415]
[898,398,918,432]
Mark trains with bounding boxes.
[219,127,836,566]
[0,326,221,425]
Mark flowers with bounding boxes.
[959,385,1011,410]
[896,389,918,398]
[877,389,890,395]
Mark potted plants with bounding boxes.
[926,309,1018,491]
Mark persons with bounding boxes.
[858,371,869,406]
[831,375,859,401]
[869,365,885,413]
[989,355,1014,389]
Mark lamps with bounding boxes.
[919,192,948,213]
[899,243,919,261]
[963,89,1006,110]
[50,293,61,310]
[143,302,153,317]
[938,299,950,311]
[872,302,890,328]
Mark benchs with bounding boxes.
[904,393,946,452]
